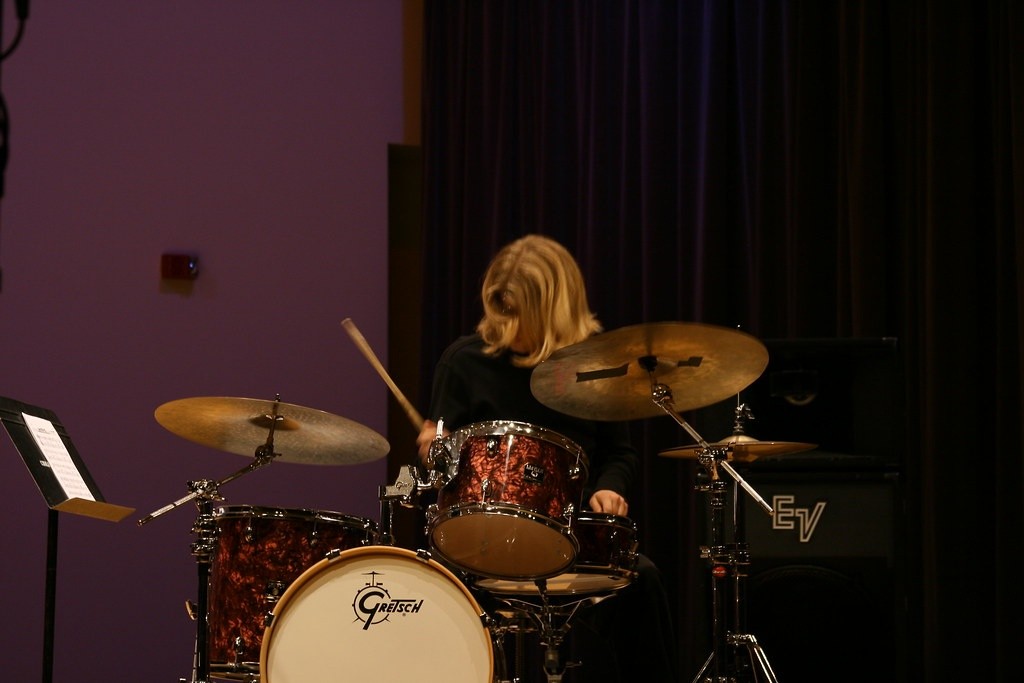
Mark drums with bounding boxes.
[206,502,382,683]
[423,419,592,583]
[257,544,496,683]
[463,511,639,601]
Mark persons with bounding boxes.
[418,234,678,683]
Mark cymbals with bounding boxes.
[152,395,391,468]
[655,434,818,465]
[528,320,770,422]
